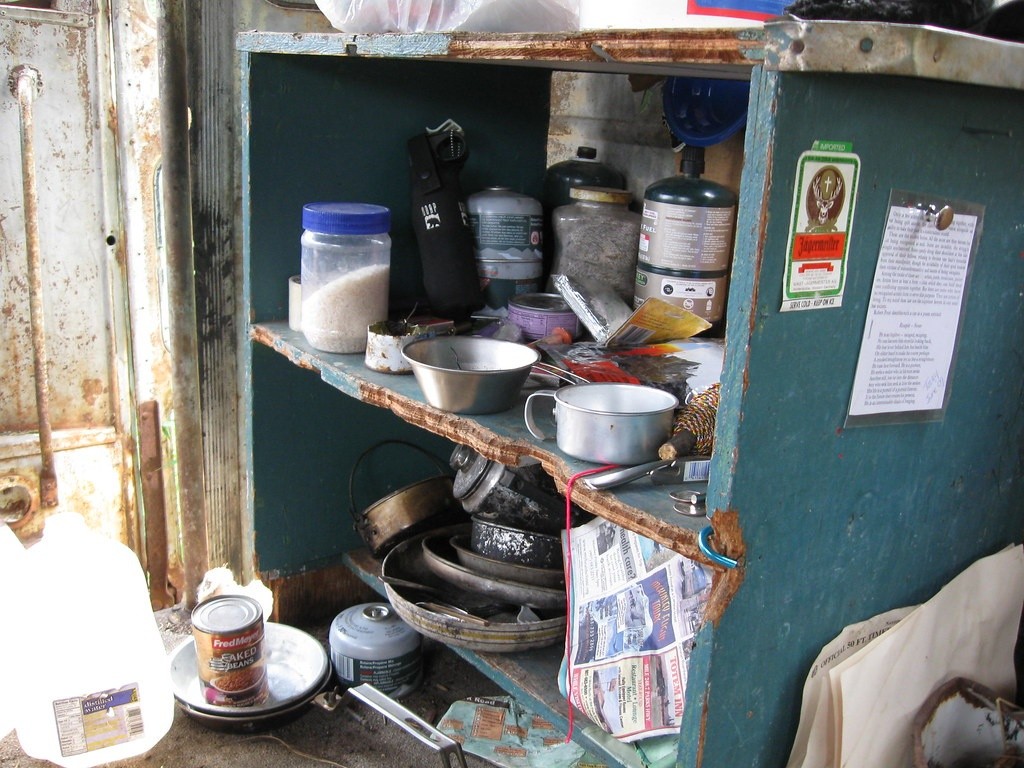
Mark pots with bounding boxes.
[524,381,680,466]
[348,440,460,559]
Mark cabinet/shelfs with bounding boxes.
[226,17,1024,768]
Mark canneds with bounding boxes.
[191,594,269,708]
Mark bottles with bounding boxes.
[468,146,735,335]
[450,443,583,536]
[0,511,175,767]
[301,201,392,354]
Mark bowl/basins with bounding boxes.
[469,515,572,573]
[401,335,542,415]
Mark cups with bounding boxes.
[289,276,309,332]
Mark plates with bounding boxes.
[165,623,332,725]
